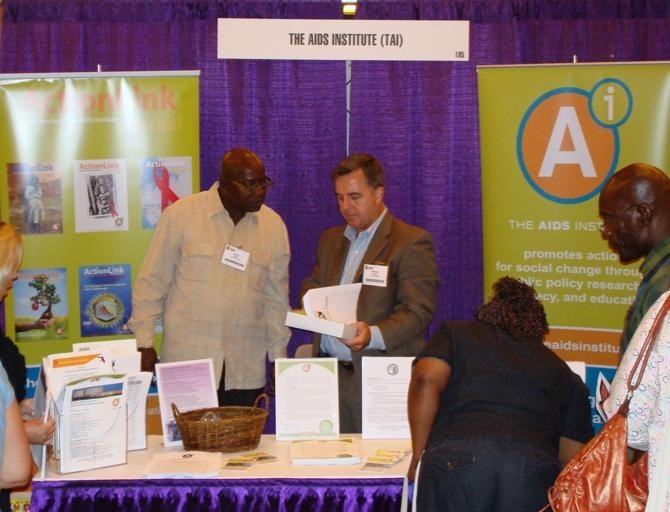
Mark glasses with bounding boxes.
[222,176,274,194]
[596,204,640,239]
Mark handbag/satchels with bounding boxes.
[547,401,649,512]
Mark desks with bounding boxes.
[28,434,422,512]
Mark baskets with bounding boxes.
[170,393,270,452]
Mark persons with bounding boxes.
[598,164,670,369]
[84,175,116,218]
[0,356,33,493]
[130,148,290,413]
[290,153,440,437]
[0,222,56,445]
[22,176,47,233]
[600,288,670,512]
[407,274,596,511]
